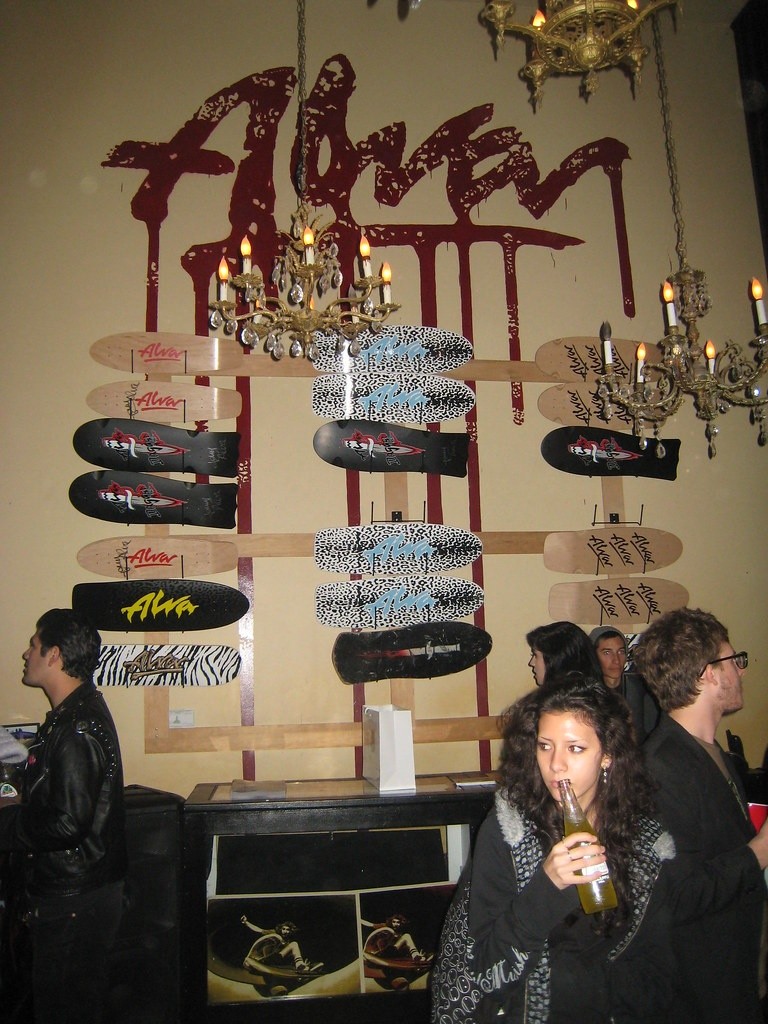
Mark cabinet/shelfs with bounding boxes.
[181,770,500,1024]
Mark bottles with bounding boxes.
[557,779,617,914]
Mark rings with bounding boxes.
[568,851,573,860]
[562,840,569,848]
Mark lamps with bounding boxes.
[480,0,686,111]
[207,0,402,360]
[597,0,768,459]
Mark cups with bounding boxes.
[747,803,768,835]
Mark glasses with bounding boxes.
[698,651,748,677]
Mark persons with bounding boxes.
[361,914,434,962]
[240,915,324,972]
[431,608,768,1024]
[18,608,124,1024]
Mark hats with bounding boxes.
[588,626,629,656]
